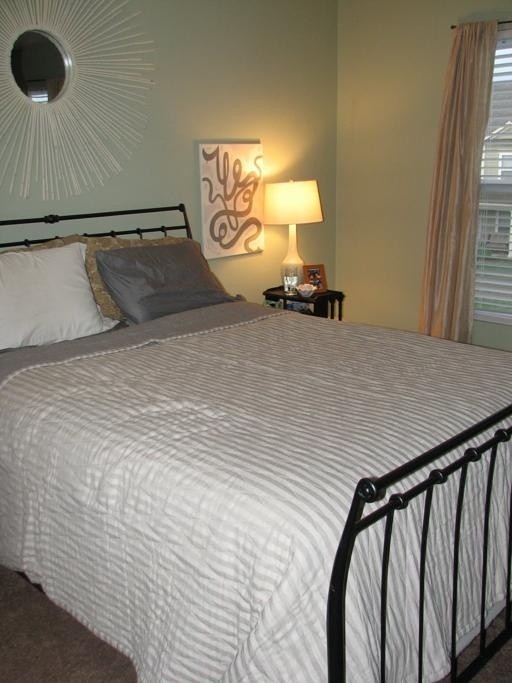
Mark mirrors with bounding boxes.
[10,30,74,106]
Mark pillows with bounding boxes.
[0,234,236,355]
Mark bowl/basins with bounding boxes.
[295,287,318,297]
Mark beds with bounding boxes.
[0,205,512,682]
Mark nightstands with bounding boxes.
[264,284,343,319]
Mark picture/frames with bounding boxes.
[301,262,326,294]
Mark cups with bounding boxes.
[282,267,298,293]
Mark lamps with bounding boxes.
[260,178,323,294]
